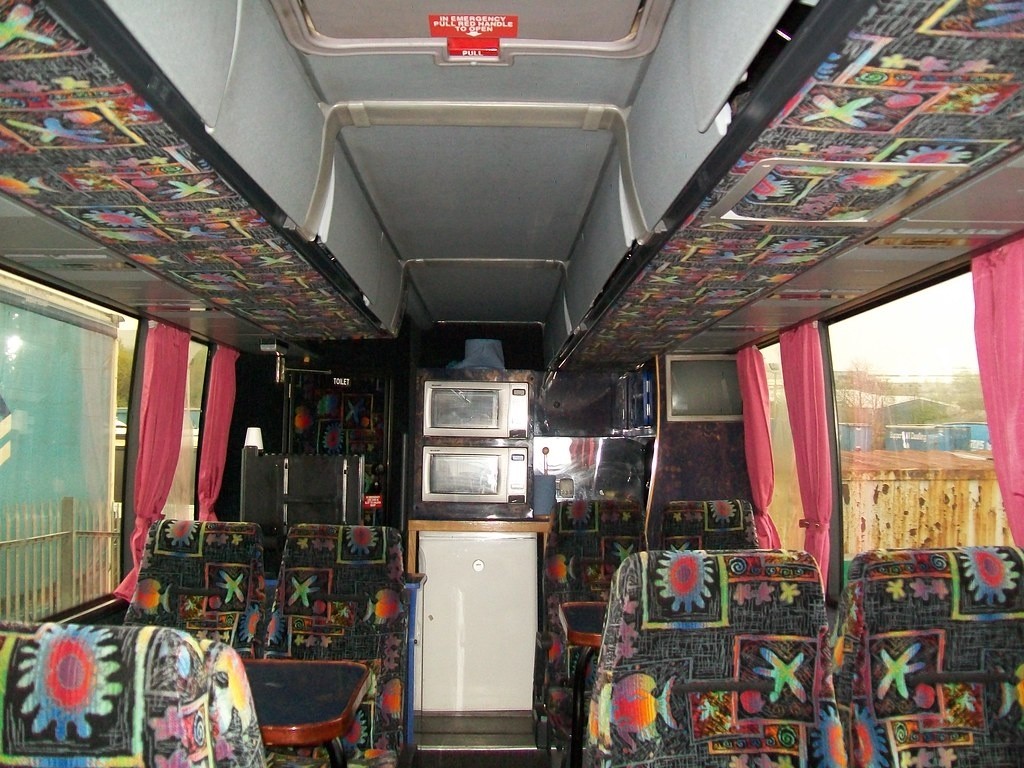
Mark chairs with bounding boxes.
[531,498,651,768]
[828,544,1024,768]
[267,523,408,768]
[581,549,850,768]
[647,500,760,548]
[122,519,265,659]
[192,637,267,768]
[0,620,215,768]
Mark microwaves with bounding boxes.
[421,446,527,505]
[422,380,528,439]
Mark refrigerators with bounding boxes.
[411,531,537,714]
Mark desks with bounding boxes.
[558,602,608,768]
[242,659,374,768]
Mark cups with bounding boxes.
[244,427,264,449]
